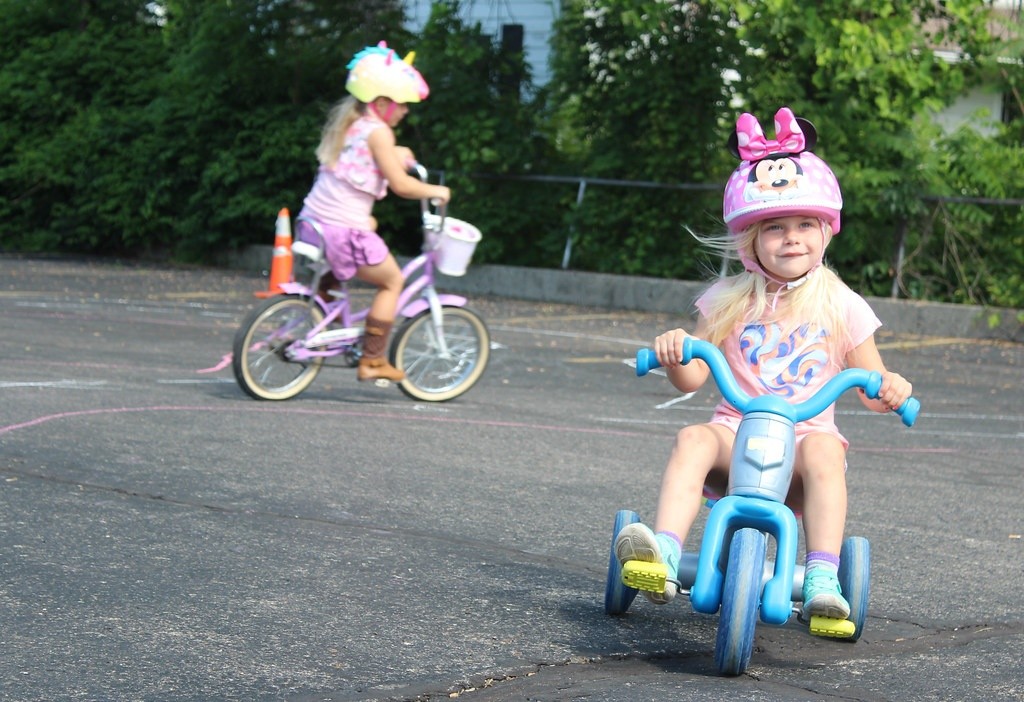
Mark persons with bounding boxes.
[613,108,913,622]
[290,41,451,381]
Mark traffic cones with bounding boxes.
[254,208,293,298]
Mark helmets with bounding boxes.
[345,41,430,105]
[721,107,843,236]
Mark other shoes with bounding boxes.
[356,356,406,380]
[310,286,342,323]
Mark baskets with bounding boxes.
[432,215,482,277]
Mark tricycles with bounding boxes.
[604,337,921,676]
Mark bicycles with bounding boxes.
[231,161,490,402]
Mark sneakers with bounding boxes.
[612,522,681,605]
[801,568,852,623]
